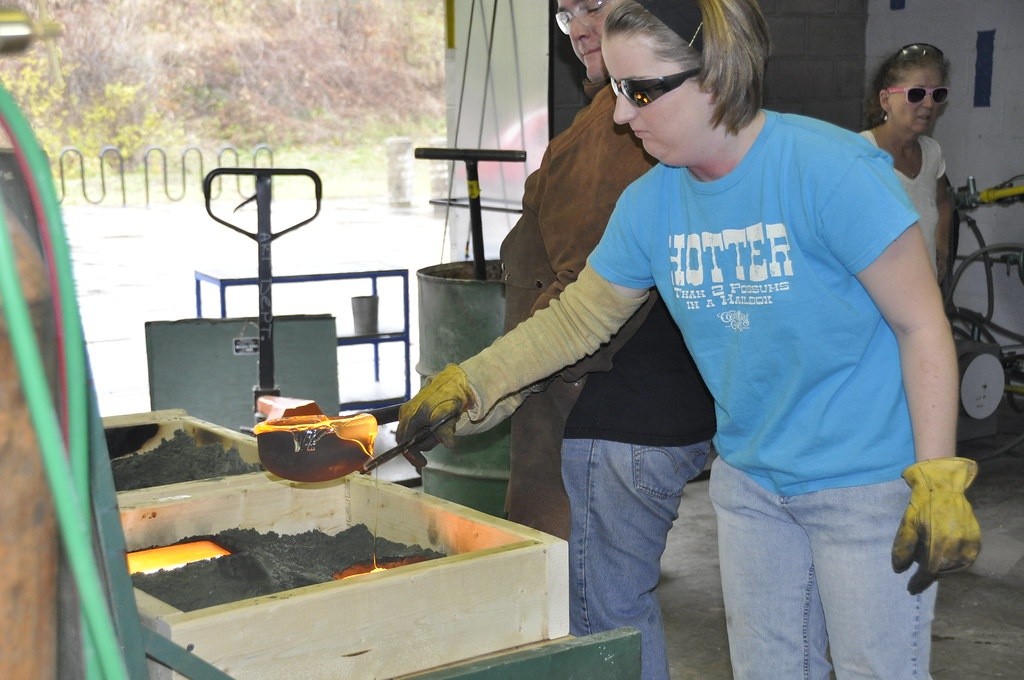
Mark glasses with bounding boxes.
[886,84,951,103]
[895,43,944,63]
[610,69,703,108]
[555,0,608,35]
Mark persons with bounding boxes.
[398,2,980,678]
[500,1,717,679]
[856,43,956,296]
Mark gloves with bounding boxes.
[891,457,983,594]
[396,363,473,468]
